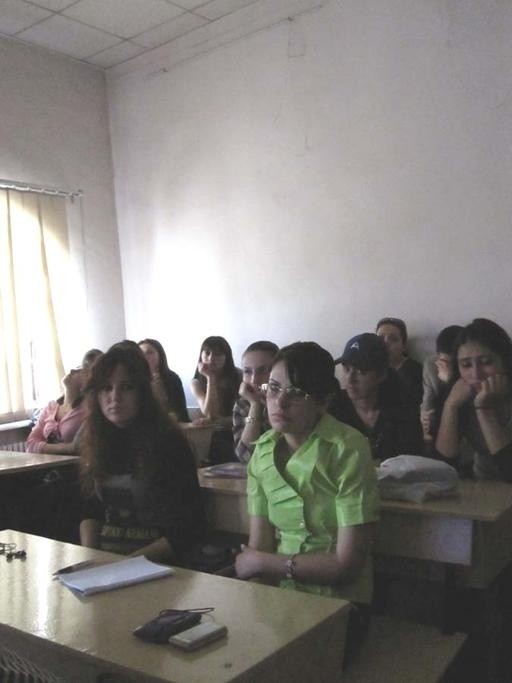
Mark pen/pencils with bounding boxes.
[52,559,96,575]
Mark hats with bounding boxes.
[333,329,394,374]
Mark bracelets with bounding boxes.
[285,552,296,579]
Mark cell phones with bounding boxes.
[168,622,228,653]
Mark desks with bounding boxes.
[177,421,225,464]
[198,461,510,628]
[0,528,351,679]
[0,450,80,523]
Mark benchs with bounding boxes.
[343,608,469,682]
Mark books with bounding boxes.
[54,552,172,594]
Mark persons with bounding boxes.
[25,362,92,464]
[138,337,195,425]
[235,340,382,663]
[57,348,105,405]
[75,339,206,569]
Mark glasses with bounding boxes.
[256,380,319,404]
